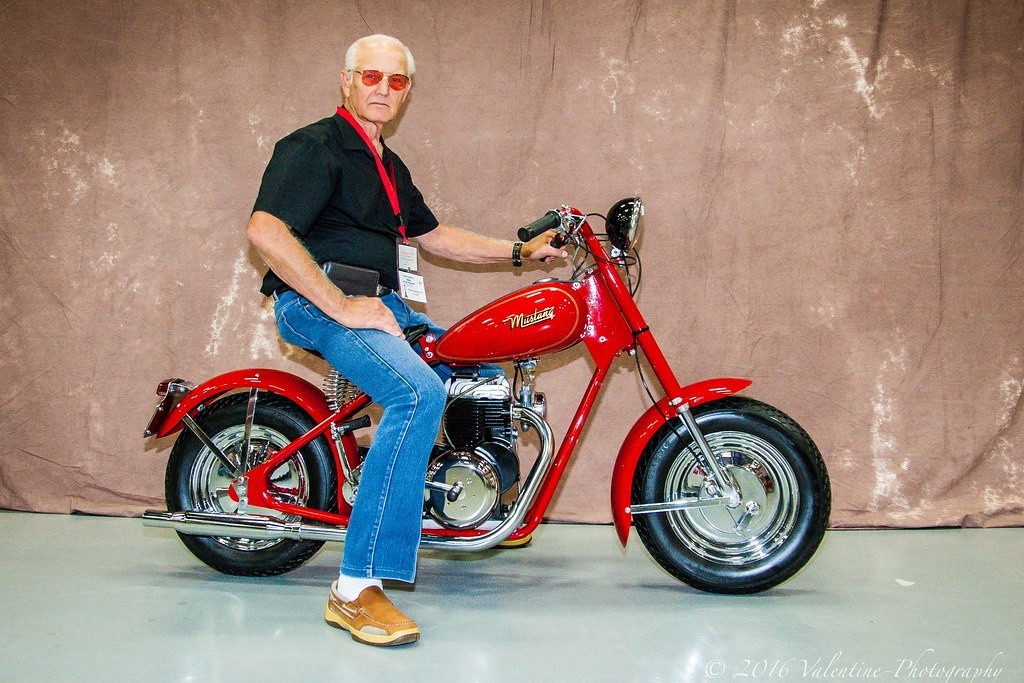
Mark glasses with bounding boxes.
[348,69,410,92]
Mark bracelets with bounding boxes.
[512,241,523,267]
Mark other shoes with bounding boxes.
[324,580,420,646]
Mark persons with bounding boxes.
[247,32,569,646]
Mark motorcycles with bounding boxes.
[136,195,834,598]
